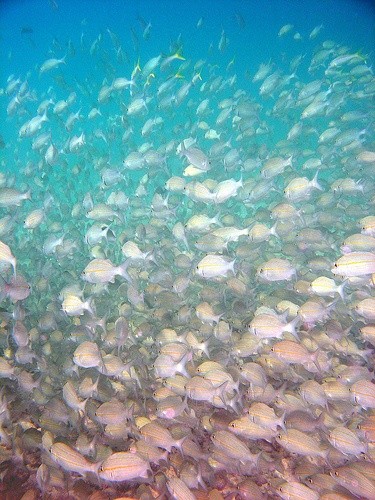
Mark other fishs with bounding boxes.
[0,0,374,500]
